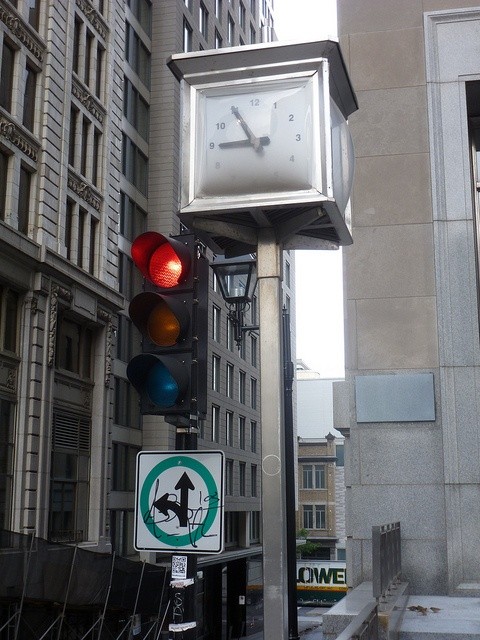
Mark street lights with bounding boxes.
[208,254,300,640]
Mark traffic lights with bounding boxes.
[126,232,208,416]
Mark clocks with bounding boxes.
[194,84,312,197]
